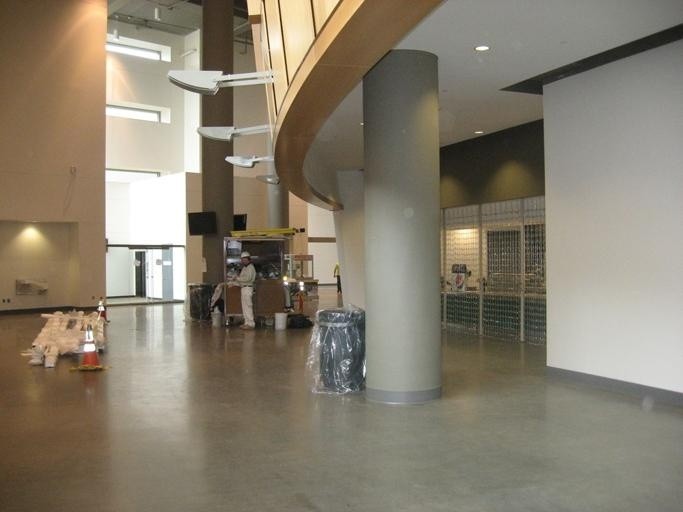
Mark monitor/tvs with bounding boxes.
[234,214,247,231]
[187,212,217,236]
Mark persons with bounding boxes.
[230,251,256,329]
[332,261,341,294]
[440,270,488,293]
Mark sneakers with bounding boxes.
[238,323,255,330]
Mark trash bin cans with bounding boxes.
[318,310,365,393]
[188,284,212,320]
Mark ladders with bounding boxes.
[230,227,305,237]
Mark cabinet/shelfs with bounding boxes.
[202,236,287,326]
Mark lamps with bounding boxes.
[166,69,281,186]
[114,15,120,41]
[154,0,162,20]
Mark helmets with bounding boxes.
[239,251,251,259]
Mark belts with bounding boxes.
[240,284,253,288]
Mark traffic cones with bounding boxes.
[97,297,111,324]
[78,324,102,372]
